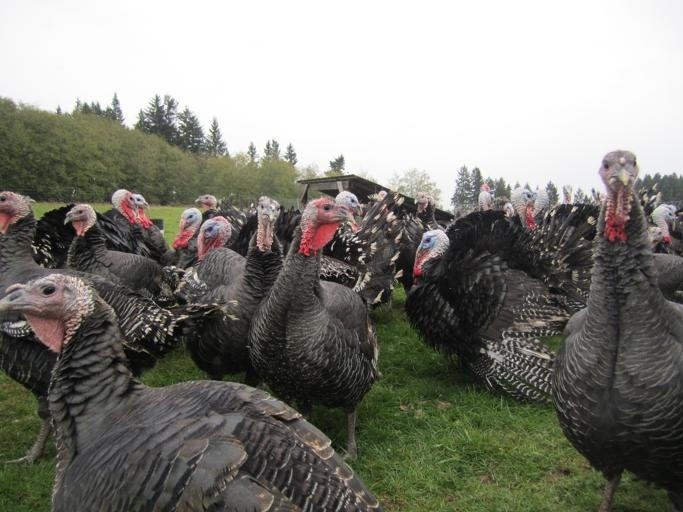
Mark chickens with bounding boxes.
[0,149,683,512]
